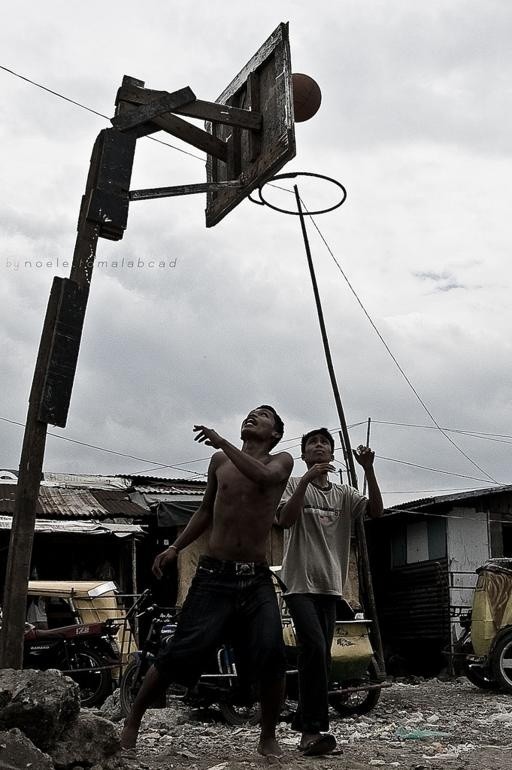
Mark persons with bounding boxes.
[121,405,294,758]
[275,428,383,756]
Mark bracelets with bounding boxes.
[169,545,178,551]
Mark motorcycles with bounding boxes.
[22,618,123,708]
[23,577,151,695]
[120,490,393,729]
[441,559,511,694]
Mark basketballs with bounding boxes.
[292,73,321,122]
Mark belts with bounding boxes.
[193,557,292,594]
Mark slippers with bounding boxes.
[120,746,140,759]
[295,733,338,757]
[265,750,290,764]
